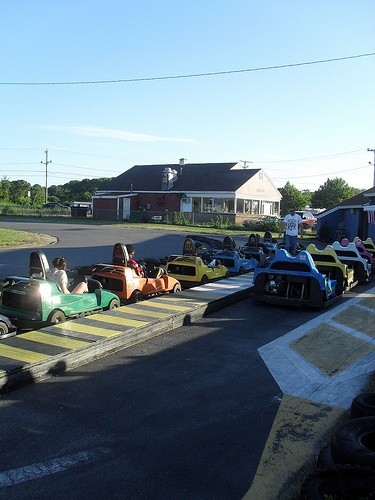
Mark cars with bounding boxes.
[243,215,279,228]
[43,203,69,209]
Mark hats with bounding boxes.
[195,243,206,250]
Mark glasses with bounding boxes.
[290,210,294,212]
[130,254,135,255]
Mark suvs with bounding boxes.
[294,211,314,221]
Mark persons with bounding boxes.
[125,244,150,278]
[47,256,88,295]
[278,207,303,256]
[195,242,219,267]
[232,233,300,260]
